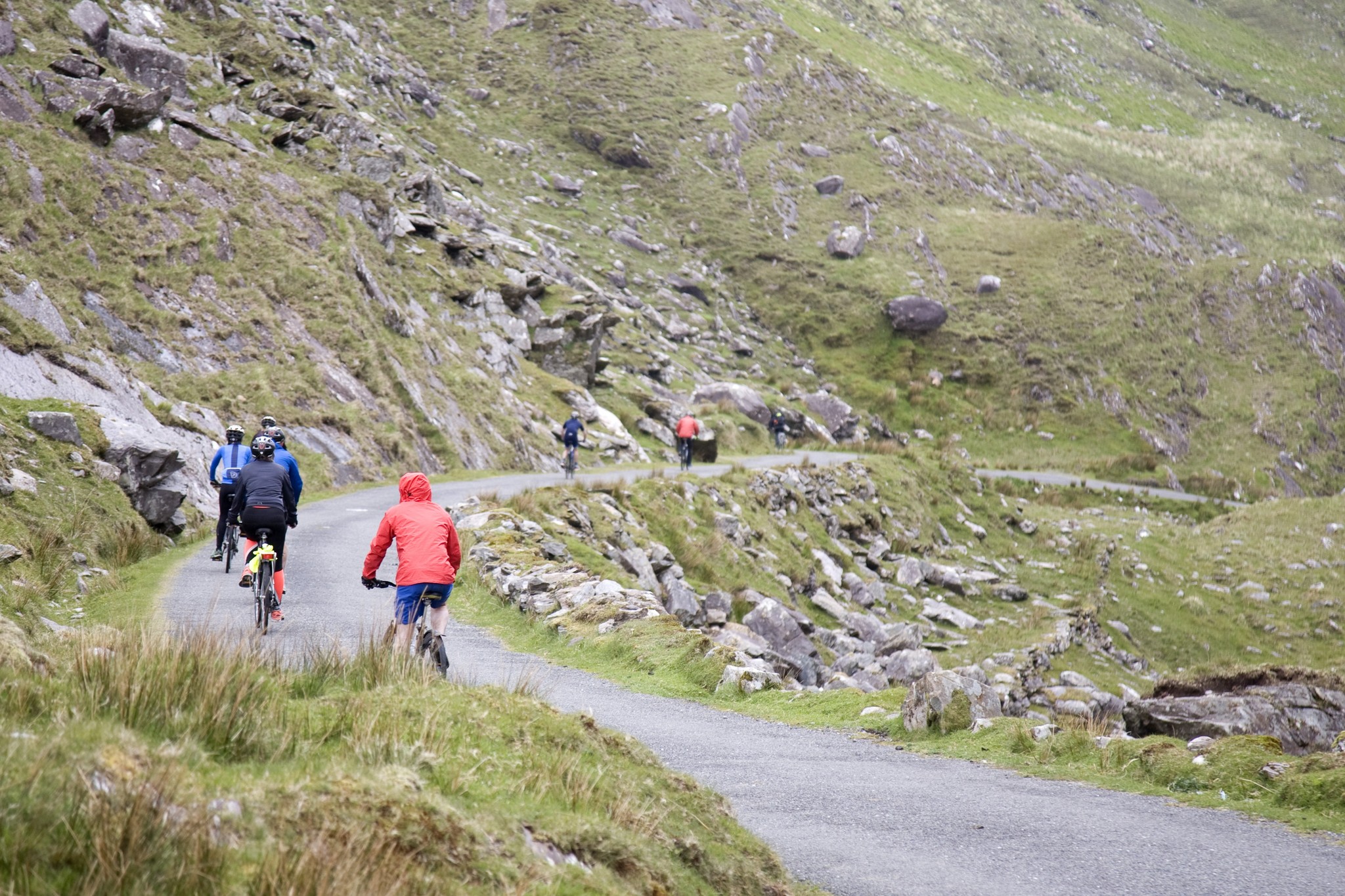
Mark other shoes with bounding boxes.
[238,566,253,587]
[272,609,284,622]
[210,549,222,558]
[434,643,450,669]
[560,463,566,469]
[574,465,580,471]
[283,585,286,591]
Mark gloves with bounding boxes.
[361,576,377,590]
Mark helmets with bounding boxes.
[686,411,694,418]
[263,426,285,443]
[570,410,580,418]
[226,425,246,440]
[261,416,277,426]
[251,436,275,457]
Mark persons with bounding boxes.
[225,436,297,623]
[210,424,255,560]
[361,472,461,672]
[246,416,303,594]
[561,411,587,468]
[675,411,700,466]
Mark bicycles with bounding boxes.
[210,482,242,574]
[771,425,787,452]
[361,580,447,681]
[227,519,296,635]
[675,433,698,471]
[561,440,585,479]
